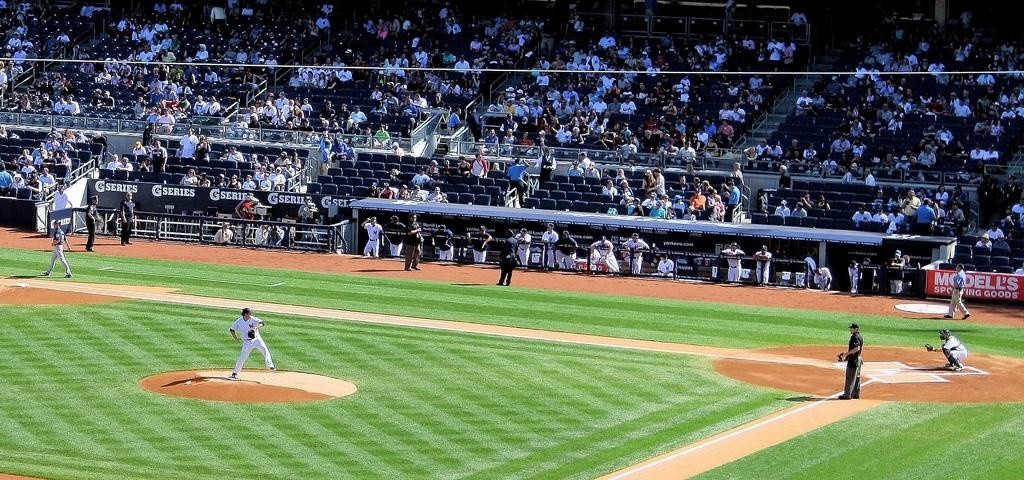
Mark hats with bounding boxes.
[895,250,901,256]
[982,233,989,240]
[849,324,858,328]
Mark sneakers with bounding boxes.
[944,315,952,318]
[962,314,970,319]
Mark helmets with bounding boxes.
[939,328,949,339]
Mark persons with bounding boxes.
[227,306,277,382]
[1,1,1023,291]
[925,327,970,375]
[835,320,865,401]
[943,264,972,323]
